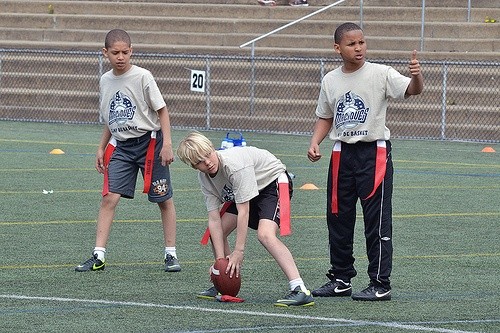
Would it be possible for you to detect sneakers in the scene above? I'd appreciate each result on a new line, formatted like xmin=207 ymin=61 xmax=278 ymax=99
xmin=352 ymin=282 xmax=391 ymax=300
xmin=197 ymin=285 xmax=221 ymax=300
xmin=165 ymin=254 xmax=180 ymax=272
xmin=311 ymin=274 xmax=352 ymax=297
xmin=274 ymin=285 xmax=314 ymax=307
xmin=75 ymin=253 xmax=105 ymax=272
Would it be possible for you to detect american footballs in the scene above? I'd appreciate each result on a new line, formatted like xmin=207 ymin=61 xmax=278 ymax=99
xmin=211 ymin=258 xmax=241 ymax=297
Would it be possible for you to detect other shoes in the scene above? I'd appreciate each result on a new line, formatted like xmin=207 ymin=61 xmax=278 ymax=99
xmin=257 ymin=0 xmax=277 ymax=6
xmin=289 ymin=0 xmax=309 ymax=7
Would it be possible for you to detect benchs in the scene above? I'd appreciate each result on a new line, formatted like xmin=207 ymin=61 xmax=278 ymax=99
xmin=0 ymin=0 xmax=500 ymax=140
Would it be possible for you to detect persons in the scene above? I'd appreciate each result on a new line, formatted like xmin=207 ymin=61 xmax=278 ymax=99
xmin=312 ymin=22 xmax=424 ymax=302
xmin=75 ymin=29 xmax=181 ymax=273
xmin=178 ymin=130 xmax=314 ymax=307
xmin=255 ymin=0 xmax=311 ymax=7
